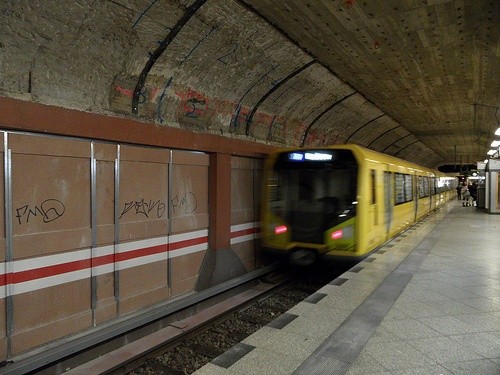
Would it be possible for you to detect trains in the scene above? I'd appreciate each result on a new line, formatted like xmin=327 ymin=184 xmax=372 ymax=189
xmin=262 ymin=144 xmax=460 ymax=267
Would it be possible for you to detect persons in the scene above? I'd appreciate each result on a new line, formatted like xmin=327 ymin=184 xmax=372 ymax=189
xmin=455 ymin=181 xmax=478 ymax=207
